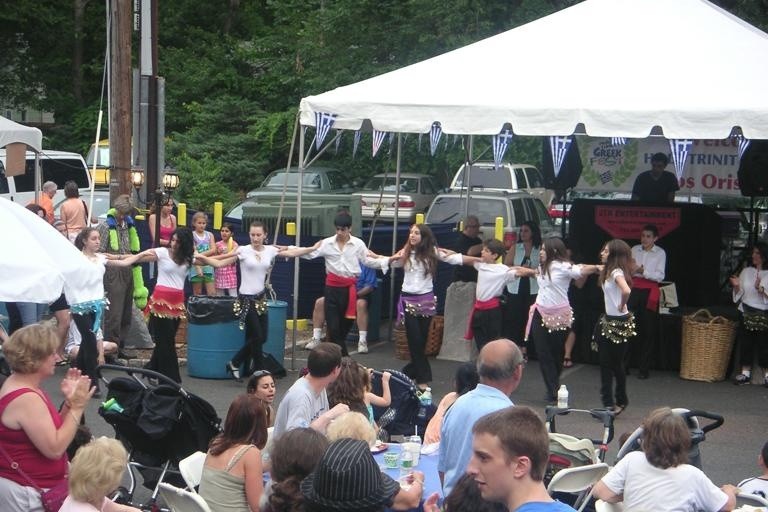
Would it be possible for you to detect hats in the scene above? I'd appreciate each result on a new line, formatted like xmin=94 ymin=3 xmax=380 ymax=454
xmin=300 ymin=439 xmax=400 ymax=510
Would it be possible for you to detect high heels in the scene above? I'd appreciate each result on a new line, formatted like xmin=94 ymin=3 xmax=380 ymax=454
xmin=142 ymin=373 xmax=158 ymax=387
xmin=226 ymin=362 xmax=242 ymax=382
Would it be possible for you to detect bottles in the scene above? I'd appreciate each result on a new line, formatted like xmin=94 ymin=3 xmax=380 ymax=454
xmin=418 ymin=385 xmax=432 ymax=417
xmin=556 ymin=384 xmax=570 ymax=409
xmin=399 ymin=449 xmax=414 ymax=476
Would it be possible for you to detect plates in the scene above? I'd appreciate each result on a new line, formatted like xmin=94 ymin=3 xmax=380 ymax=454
xmin=370 ymin=443 xmax=390 ymax=453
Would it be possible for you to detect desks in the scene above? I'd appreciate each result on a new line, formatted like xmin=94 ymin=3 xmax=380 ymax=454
xmin=356 ymin=439 xmax=448 ymax=511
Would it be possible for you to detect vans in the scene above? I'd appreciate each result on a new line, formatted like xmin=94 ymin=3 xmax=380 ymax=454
xmin=83 ymin=137 xmax=135 ymax=189
xmin=0 ymin=147 xmax=96 ymax=226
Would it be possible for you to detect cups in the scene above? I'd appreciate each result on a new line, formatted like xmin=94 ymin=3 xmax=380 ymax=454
xmin=402 ymin=435 xmax=422 ymax=467
xmin=103 ymin=398 xmax=124 ymax=415
xmin=383 ymin=451 xmax=400 ymax=468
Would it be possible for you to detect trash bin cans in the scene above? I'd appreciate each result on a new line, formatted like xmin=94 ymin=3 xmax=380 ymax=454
xmin=188 ymin=296 xmax=288 ymax=379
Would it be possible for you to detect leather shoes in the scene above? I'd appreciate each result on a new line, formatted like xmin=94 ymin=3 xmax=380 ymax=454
xmin=522 ymin=357 xmax=767 ymax=417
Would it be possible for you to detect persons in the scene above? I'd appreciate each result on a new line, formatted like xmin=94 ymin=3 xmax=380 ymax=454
xmin=624 ymin=225 xmax=666 ymax=379
xmin=730 ymin=245 xmax=767 ymax=385
xmin=196 ymin=214 xmax=635 ymax=411
xmin=1 ymin=322 xmax=767 ymax=511
xmin=631 ymin=152 xmax=680 ymax=202
xmin=26 ymin=179 xmax=241 ymax=396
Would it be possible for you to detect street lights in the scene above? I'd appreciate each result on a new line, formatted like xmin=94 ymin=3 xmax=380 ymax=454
xmin=128 ymin=169 xmax=181 ymax=284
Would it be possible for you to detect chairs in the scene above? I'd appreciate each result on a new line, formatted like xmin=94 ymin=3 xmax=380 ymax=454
xmin=155 ymin=478 xmax=215 ymax=510
xmin=545 ymin=463 xmax=608 ymax=512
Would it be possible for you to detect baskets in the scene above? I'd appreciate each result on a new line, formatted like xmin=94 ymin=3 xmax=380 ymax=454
xmin=390 ymin=315 xmax=444 ymax=359
xmin=680 ymin=308 xmax=737 ymax=382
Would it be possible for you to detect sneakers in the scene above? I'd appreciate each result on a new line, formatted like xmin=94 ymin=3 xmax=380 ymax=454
xmin=357 ymin=341 xmax=368 ymax=353
xmin=305 ymin=339 xmax=320 ymax=350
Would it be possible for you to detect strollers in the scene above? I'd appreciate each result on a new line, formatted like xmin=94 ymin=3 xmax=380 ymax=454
xmin=367 ymin=366 xmax=441 ymax=445
xmin=93 ymin=360 xmax=229 ymax=511
xmin=542 ymin=403 xmax=625 ymax=509
xmin=610 ymin=407 xmax=732 ymax=470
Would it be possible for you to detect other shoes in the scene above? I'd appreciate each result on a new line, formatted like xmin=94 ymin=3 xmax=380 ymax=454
xmin=90 ymin=380 xmax=101 ymax=396
xmin=419 ymin=385 xmax=430 ymax=393
